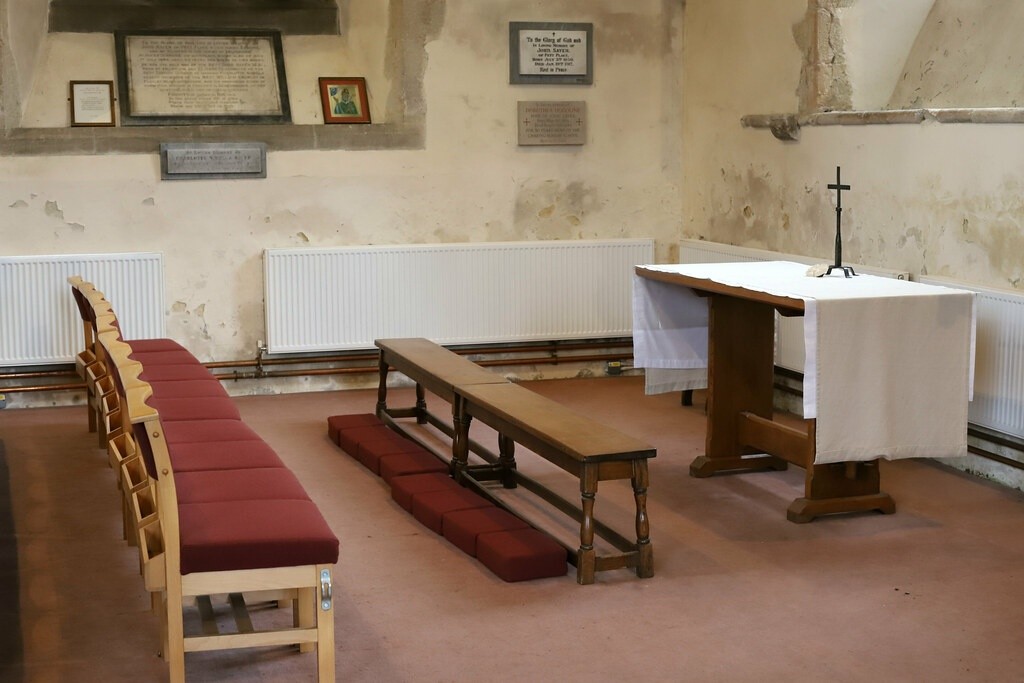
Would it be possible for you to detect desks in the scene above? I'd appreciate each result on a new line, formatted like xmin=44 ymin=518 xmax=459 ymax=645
xmin=631 ymin=260 xmax=976 ymax=524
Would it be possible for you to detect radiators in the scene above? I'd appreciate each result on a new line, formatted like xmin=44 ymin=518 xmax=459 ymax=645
xmin=0 ymin=252 xmax=166 ymax=367
xmin=678 ymin=238 xmax=909 ymax=375
xmin=918 ymin=275 xmax=1024 ymax=439
xmin=262 ymin=238 xmax=655 ymax=353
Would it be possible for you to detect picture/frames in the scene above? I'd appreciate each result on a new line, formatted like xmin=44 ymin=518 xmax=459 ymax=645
xmin=70 ymin=80 xmax=115 ymax=127
xmin=114 ymin=28 xmax=293 ymax=126
xmin=508 ymin=21 xmax=593 ymax=85
xmin=319 ymin=76 xmax=371 ymax=124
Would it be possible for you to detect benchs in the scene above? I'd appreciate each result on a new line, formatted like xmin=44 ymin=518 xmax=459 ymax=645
xmin=374 ymin=338 xmax=656 ymax=586
xmin=68 ymin=273 xmax=341 ymax=683
